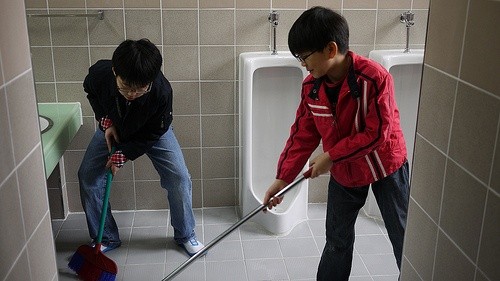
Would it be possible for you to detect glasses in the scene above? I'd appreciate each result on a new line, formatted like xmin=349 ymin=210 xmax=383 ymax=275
xmin=115 ymin=68 xmax=152 ymax=94
xmin=295 ymin=38 xmax=337 ymax=63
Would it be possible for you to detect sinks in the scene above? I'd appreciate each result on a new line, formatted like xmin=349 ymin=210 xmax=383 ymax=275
xmin=39 ymin=116 xmax=49 ymax=132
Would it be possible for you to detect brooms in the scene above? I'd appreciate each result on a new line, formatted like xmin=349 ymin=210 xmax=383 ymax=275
xmin=67 ymin=146 xmax=118 ymax=281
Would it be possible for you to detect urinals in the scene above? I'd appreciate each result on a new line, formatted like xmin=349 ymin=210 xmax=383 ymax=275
xmin=239 ymin=51 xmax=310 ymax=234
xmin=362 ymin=49 xmax=425 ymax=221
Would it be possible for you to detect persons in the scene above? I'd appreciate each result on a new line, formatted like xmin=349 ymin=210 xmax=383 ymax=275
xmin=77 ymin=38 xmax=209 ymax=259
xmin=262 ymin=6 xmax=411 ymax=281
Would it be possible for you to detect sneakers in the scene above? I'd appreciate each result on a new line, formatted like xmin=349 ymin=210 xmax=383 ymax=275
xmin=88 ymin=240 xmax=119 ymax=253
xmin=177 ymin=235 xmax=208 ymax=257
